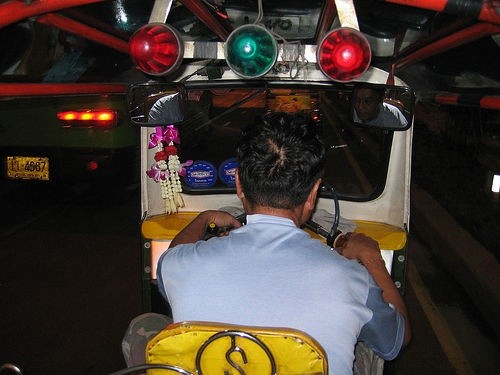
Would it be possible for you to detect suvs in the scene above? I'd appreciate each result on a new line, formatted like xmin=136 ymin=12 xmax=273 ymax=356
xmin=0 ymin=28 xmax=162 ymax=192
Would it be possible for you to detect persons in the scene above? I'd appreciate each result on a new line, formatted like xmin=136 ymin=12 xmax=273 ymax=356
xmin=352 ymin=85 xmax=407 ymax=127
xmin=148 ymin=86 xmax=203 ymax=125
xmin=156 ymin=112 xmax=411 ymax=375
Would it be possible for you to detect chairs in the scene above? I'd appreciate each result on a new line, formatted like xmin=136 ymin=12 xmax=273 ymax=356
xmin=146 ymin=321 xmax=329 ymax=374
